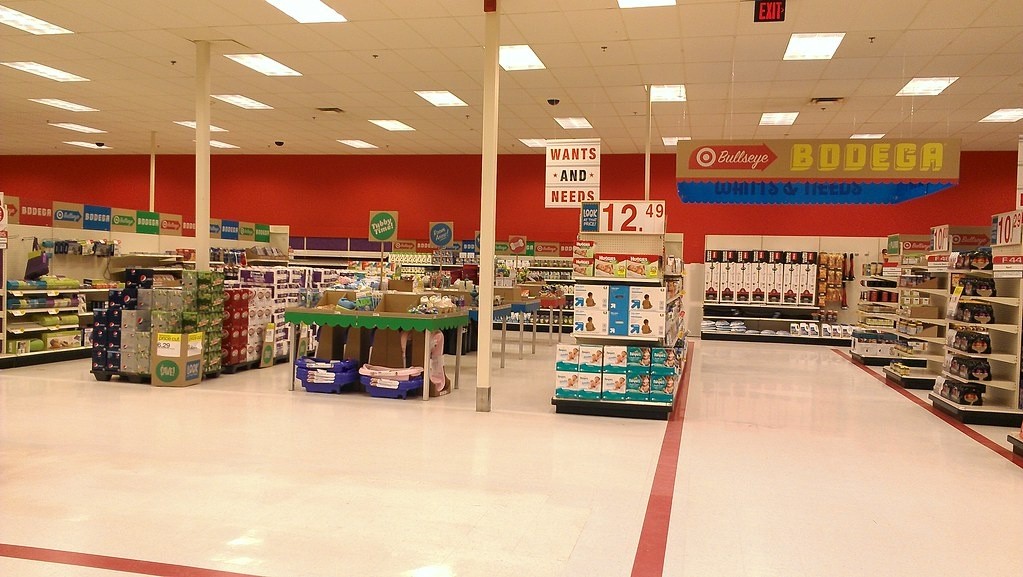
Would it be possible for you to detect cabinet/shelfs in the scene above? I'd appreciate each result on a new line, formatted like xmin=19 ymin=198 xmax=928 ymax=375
xmin=850 ymin=210 xmax=1023 ymax=459
xmin=702 ymin=232 xmax=892 ymax=347
xmin=0 ymin=191 xmax=291 ymax=359
xmin=551 ymin=224 xmax=690 ymax=422
xmin=302 ymin=240 xmax=577 ymax=332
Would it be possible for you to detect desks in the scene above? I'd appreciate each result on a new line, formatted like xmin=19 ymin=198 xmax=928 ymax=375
xmin=282 ymin=281 xmax=568 ymax=401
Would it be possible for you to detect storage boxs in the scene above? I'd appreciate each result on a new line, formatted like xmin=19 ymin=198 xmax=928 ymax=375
xmin=92 ymin=266 xmax=368 ymax=389
xmin=552 ymin=241 xmax=678 ymax=404
xmin=704 ymin=250 xmax=817 ymax=306
